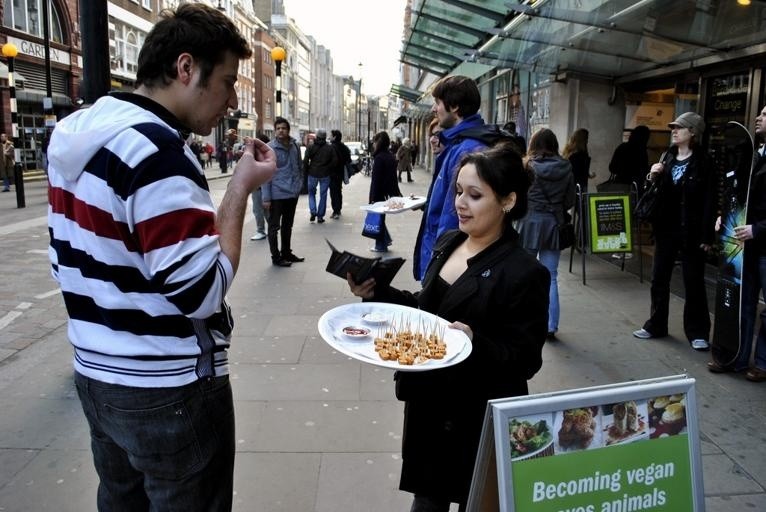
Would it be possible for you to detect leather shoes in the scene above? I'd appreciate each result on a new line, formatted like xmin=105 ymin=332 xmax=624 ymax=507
xmin=273 ymin=259 xmax=292 ymax=267
xmin=283 ymin=254 xmax=304 ymax=261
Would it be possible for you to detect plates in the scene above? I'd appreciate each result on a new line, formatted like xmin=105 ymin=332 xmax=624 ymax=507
xmin=318 ymin=302 xmax=474 ymax=372
xmin=342 ymin=325 xmax=371 ymax=340
xmin=361 ymin=312 xmax=388 ymax=325
xmin=361 ymin=197 xmax=427 ymax=213
xmin=604 ymin=427 xmax=657 ymax=447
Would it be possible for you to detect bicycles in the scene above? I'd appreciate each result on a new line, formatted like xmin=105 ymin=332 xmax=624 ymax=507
xmin=359 ymin=147 xmax=375 ymax=176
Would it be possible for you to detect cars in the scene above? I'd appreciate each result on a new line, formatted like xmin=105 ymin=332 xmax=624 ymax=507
xmin=18 ymin=137 xmax=41 ymax=160
xmin=297 ymin=139 xmax=365 ymax=193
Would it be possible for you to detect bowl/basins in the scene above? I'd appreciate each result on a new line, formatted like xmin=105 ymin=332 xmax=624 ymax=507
xmin=649 ymin=409 xmax=685 ymax=438
xmin=512 ymin=425 xmax=555 ymax=462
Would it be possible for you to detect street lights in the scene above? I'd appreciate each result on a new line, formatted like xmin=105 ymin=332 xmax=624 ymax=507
xmin=1 ymin=41 xmax=29 ymax=209
xmin=272 ymin=44 xmax=284 ymax=121
xmin=357 ymin=58 xmax=365 ymax=139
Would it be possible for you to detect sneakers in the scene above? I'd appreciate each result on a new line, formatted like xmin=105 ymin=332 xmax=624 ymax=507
xmin=691 ymin=339 xmax=709 ymax=350
xmin=369 ymin=241 xmax=392 ymax=252
xmin=611 ymin=253 xmax=633 ymax=260
xmin=250 ymin=233 xmax=266 ymax=240
xmin=310 ymin=213 xmax=339 ymax=223
xmin=707 ymin=360 xmax=748 ymax=374
xmin=633 ymin=327 xmax=652 ymax=339
xmin=745 ymin=366 xmax=766 ymax=382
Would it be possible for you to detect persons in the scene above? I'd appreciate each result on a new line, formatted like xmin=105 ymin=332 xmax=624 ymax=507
xmin=347 ymin=146 xmax=551 ymax=512
xmin=45 ymin=4 xmax=277 ymax=512
xmin=41 ymin=128 xmax=51 ymax=175
xmin=0 ymin=134 xmax=16 ymax=191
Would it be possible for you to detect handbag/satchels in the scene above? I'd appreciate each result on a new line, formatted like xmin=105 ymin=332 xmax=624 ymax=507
xmin=343 ymin=165 xmax=349 ymax=184
xmin=632 ymin=186 xmax=659 ymax=232
xmin=362 ymin=213 xmax=386 ymax=241
xmin=548 ymin=224 xmax=576 ymax=251
xmin=596 ymin=180 xmax=634 ymax=193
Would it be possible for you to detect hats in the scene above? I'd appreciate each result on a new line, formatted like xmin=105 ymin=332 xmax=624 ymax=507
xmin=667 ymin=112 xmax=706 ymax=137
xmin=316 ymin=128 xmax=327 ymax=138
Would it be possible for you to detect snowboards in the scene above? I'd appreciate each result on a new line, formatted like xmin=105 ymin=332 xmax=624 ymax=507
xmin=712 ymin=120 xmax=754 ymax=367
